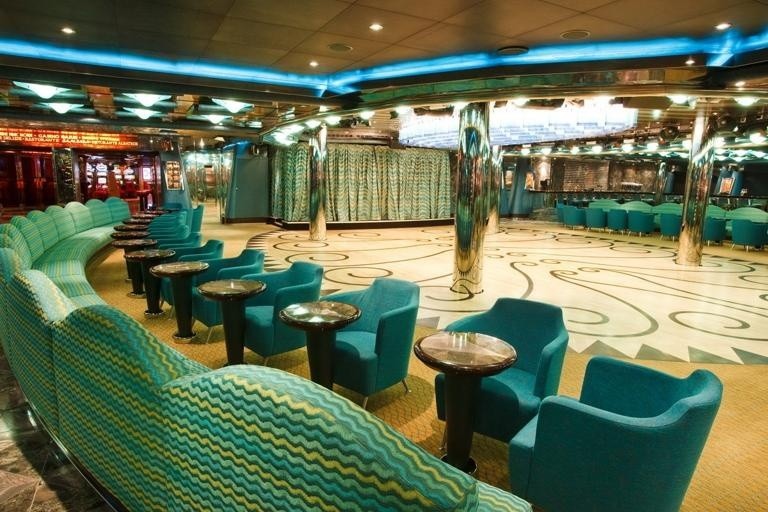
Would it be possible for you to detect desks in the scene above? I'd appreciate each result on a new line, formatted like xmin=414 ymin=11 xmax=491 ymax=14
xmin=276 ymin=299 xmax=361 ymax=400
xmin=412 ymin=326 xmax=515 ymax=481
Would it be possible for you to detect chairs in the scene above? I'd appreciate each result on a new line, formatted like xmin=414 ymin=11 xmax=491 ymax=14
xmin=50 ymin=297 xmax=216 ymax=500
xmin=1 ymin=192 xmax=341 ymax=386
xmin=434 ymin=299 xmax=725 ymax=510
xmin=319 ymin=275 xmax=420 ymax=410
xmin=2 ymin=269 xmax=116 ymax=473
xmin=168 ymin=344 xmax=543 ymax=512
xmin=551 ymin=192 xmax=767 ymax=253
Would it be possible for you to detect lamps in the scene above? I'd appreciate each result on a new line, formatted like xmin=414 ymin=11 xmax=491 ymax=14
xmin=116 ymin=87 xmax=172 ymax=122
xmin=194 ymin=93 xmax=257 ymax=125
xmin=397 ymin=93 xmax=768 ymax=164
xmin=16 ymin=77 xmax=84 ymax=120
xmin=273 ymin=108 xmax=376 ymax=147
